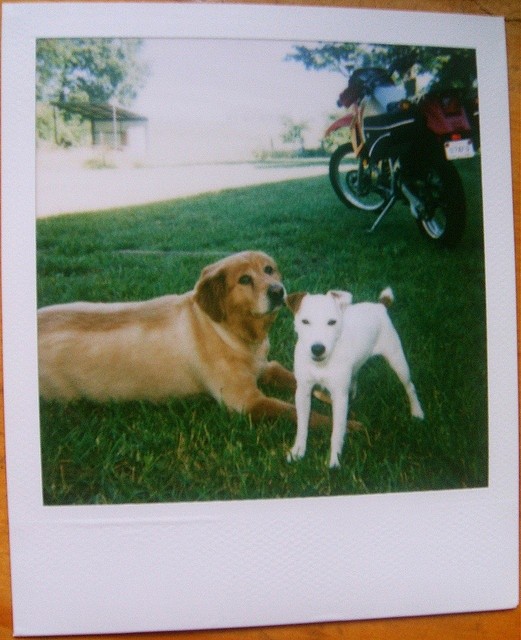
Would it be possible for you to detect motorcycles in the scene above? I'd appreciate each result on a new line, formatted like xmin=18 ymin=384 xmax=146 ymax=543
xmin=324 ymin=50 xmax=476 ymax=241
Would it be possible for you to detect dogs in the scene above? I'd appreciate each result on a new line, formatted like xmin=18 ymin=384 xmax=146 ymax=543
xmin=281 ymin=285 xmax=423 ymax=469
xmin=35 ymin=249 xmax=362 ymax=434
